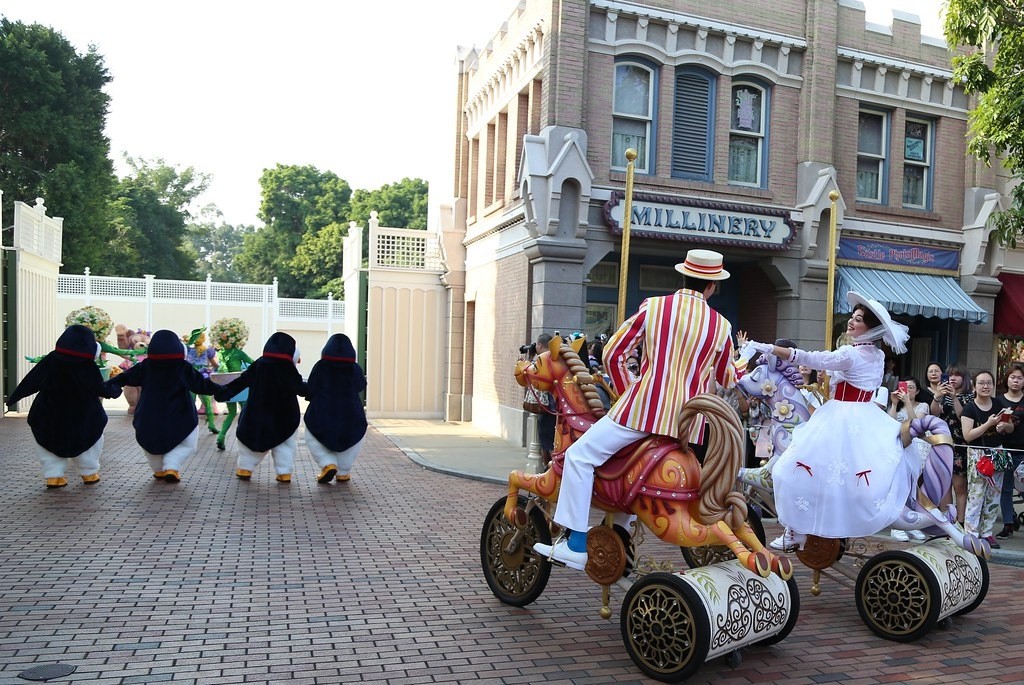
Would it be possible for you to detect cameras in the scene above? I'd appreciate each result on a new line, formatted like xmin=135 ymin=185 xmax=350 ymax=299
xmin=628 ymin=362 xmax=639 ymax=370
xmin=520 ymin=342 xmax=537 ymax=355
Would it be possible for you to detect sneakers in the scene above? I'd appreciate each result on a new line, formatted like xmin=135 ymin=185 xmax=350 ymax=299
xmin=890 ymin=527 xmax=910 ymax=542
xmin=906 ymin=527 xmax=926 ymax=540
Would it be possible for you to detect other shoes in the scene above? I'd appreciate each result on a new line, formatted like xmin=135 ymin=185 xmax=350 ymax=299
xmin=997 ymin=526 xmax=1014 ymax=539
xmin=985 ymin=534 xmax=1000 ymax=550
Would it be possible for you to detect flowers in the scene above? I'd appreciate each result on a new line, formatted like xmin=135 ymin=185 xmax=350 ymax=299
xmin=65 ymin=306 xmax=115 ymax=343
xmin=209 ymin=318 xmax=249 ymax=350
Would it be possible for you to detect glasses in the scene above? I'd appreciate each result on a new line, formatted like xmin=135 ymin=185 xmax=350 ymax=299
xmin=977 ymin=381 xmax=994 ymax=386
xmin=908 ymin=385 xmax=917 ymax=389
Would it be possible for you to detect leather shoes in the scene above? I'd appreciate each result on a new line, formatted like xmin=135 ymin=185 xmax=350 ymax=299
xmin=534 ymin=539 xmax=588 ymax=570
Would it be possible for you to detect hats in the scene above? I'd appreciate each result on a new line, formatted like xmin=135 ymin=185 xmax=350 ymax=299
xmin=676 ymin=250 xmax=729 ymax=280
xmin=847 ymin=291 xmax=910 ymax=355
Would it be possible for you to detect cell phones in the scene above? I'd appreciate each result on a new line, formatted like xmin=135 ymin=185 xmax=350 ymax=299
xmin=941 ymin=372 xmax=949 ymax=386
xmin=898 ymin=381 xmax=908 ymax=394
xmin=996 ymin=409 xmax=1006 ymax=416
xmin=555 ymin=330 xmax=560 ymax=335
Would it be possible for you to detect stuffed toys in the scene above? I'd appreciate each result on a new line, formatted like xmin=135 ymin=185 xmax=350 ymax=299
xmin=215 ymin=332 xmax=307 ymax=481
xmin=6 ymin=324 xmax=123 ymax=489
xmin=114 ymin=324 xmax=152 ymax=414
xmin=303 ymin=333 xmax=368 ymax=482
xmin=108 ymin=329 xmax=230 ymax=484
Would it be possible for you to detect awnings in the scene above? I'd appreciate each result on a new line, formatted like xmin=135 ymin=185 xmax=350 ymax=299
xmin=835 ymin=265 xmax=988 ymax=325
xmin=995 ymin=272 xmax=1024 ymax=344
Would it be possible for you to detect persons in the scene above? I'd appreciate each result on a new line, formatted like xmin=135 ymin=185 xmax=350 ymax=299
xmin=882 ymin=358 xmax=899 ymax=393
xmin=995 ymin=365 xmax=1024 ymax=539
xmin=959 ymin=371 xmax=1014 ymax=550
xmin=887 ymin=377 xmax=929 ymax=541
xmin=915 ymin=363 xmax=947 ymax=404
xmin=525 ymin=331 xmax=643 ymax=473
xmin=531 ymin=248 xmax=738 ymax=570
xmin=740 ymin=290 xmax=922 ymax=550
xmin=930 ymin=363 xmax=978 ymax=528
xmin=688 ymin=330 xmax=825 ymax=469
xmin=24 ymin=325 xmax=255 ymax=451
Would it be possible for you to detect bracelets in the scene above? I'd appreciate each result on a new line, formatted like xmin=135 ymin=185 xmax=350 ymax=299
xmin=933 ymin=395 xmax=942 ymax=402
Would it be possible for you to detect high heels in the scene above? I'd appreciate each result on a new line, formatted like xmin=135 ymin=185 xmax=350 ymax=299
xmin=769 ymin=527 xmax=808 ymax=551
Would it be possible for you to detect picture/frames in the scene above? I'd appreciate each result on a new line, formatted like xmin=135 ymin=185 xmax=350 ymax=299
xmin=586 ymin=303 xmax=617 ymax=342
xmin=639 ymin=264 xmax=685 ymax=291
xmin=587 ymin=261 xmax=618 ymax=288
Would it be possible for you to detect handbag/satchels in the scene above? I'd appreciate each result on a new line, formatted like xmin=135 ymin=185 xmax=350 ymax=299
xmin=985 ymin=449 xmax=1014 ymax=472
